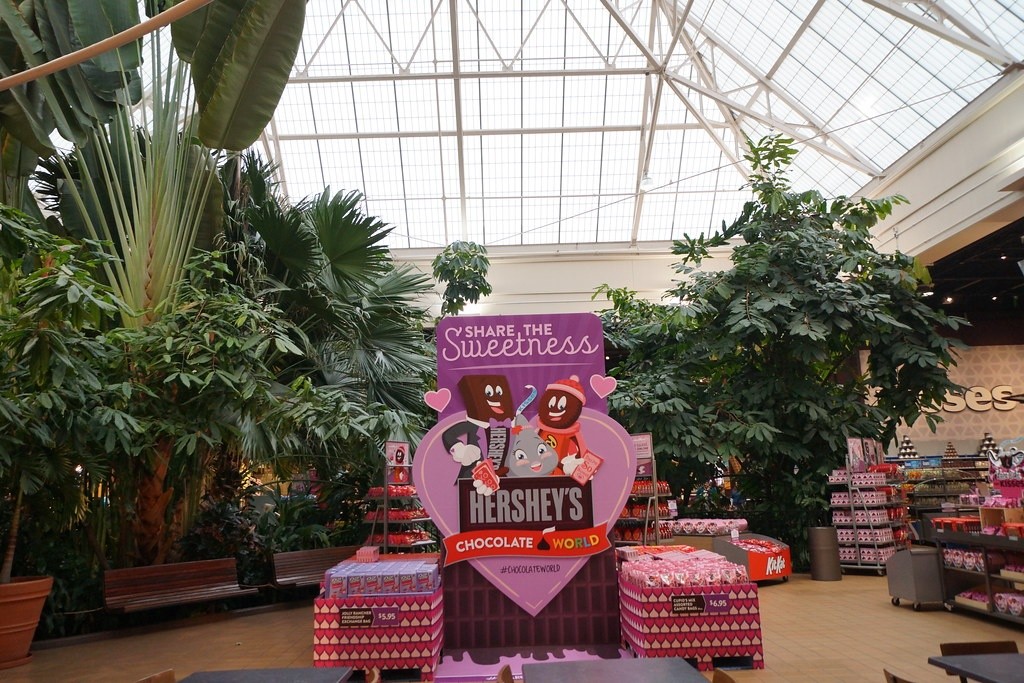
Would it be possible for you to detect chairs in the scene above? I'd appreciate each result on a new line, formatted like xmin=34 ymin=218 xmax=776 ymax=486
xmin=940 ymin=641 xmax=1019 ymax=683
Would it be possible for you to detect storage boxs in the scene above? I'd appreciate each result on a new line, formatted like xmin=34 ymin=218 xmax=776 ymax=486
xmin=325 ymin=547 xmax=441 ymax=598
xmin=829 ymin=463 xmax=895 ymax=561
xmin=979 ymin=507 xmax=1023 ymax=529
xmin=942 ymin=548 xmax=1005 ymax=576
xmin=615 ymin=546 xmax=726 ymax=562
xmin=955 ymin=583 xmax=1024 ymax=616
xmin=1000 ymin=569 xmax=1024 ymax=581
xmin=651 ymin=518 xmax=747 ymax=534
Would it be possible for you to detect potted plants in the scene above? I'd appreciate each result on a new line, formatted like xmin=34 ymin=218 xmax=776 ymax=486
xmin=0 ymin=251 xmax=271 ymax=670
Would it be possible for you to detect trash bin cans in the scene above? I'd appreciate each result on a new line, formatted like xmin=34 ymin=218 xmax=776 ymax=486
xmin=806 ymin=526 xmax=843 ymax=582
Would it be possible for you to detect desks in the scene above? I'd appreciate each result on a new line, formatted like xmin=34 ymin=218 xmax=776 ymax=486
xmin=178 ymin=667 xmax=352 ymax=683
xmin=522 ymin=657 xmax=712 ymax=683
xmin=928 ymin=654 xmax=1024 ymax=683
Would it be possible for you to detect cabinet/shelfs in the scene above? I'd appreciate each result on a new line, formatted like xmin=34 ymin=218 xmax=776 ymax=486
xmin=936 ymin=529 xmax=1024 ymax=624
xmin=827 ymin=455 xmax=891 ymax=575
xmin=887 ymin=455 xmax=989 ymax=544
xmin=673 ymin=531 xmax=752 ymax=551
xmin=313 ymin=585 xmax=446 ymax=683
xmin=614 ymin=452 xmax=673 ymax=546
xmin=615 ymin=570 xmax=764 ymax=671
xmin=363 ymin=465 xmax=436 ymax=554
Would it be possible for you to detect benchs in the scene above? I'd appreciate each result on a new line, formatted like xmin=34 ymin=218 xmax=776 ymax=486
xmin=104 ymin=558 xmax=258 ymax=612
xmin=273 ymin=545 xmax=362 ymax=585
